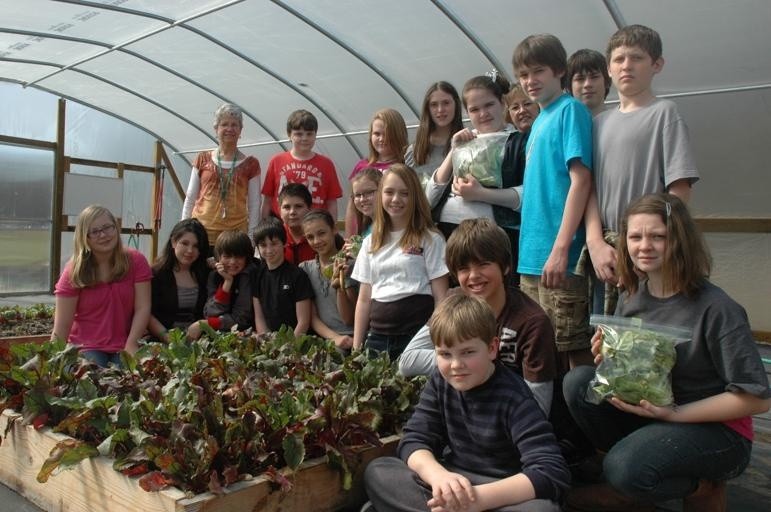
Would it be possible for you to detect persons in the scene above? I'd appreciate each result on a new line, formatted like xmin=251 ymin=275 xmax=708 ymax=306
xmin=361 ymin=293 xmax=571 ymax=511
xmin=563 ymin=194 xmax=771 ymax=511
xmin=19 ymin=204 xmax=152 ymax=369
xmin=145 ymin=26 xmax=699 ymax=457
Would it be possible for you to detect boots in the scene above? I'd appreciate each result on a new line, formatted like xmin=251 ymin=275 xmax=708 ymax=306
xmin=683 ymin=479 xmax=726 ymax=512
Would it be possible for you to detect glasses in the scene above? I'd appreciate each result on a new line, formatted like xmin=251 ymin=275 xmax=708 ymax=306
xmin=87 ymin=224 xmax=116 ymax=238
xmin=351 ymin=190 xmax=376 ymax=201
